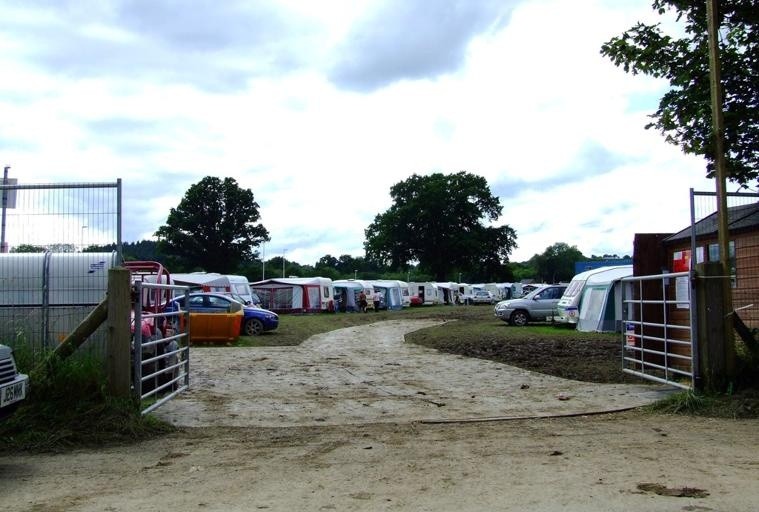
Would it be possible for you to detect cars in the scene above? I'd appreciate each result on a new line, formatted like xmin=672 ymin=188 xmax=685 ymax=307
xmin=473 ymin=291 xmax=495 ymax=305
xmin=494 ymin=285 xmax=568 ymax=326
xmin=161 ymin=293 xmax=279 ymax=336
xmin=0 ymin=344 xmax=31 ymax=423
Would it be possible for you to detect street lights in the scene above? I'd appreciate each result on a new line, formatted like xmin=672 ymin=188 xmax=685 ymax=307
xmin=283 ymin=248 xmax=288 ymax=278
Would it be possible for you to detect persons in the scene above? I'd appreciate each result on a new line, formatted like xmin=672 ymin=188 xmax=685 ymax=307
xmin=360 ymin=289 xmax=367 ymax=313
xmin=372 ymin=289 xmax=383 ymax=311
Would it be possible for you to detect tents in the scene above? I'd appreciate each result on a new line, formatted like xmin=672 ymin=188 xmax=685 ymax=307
xmin=432 ymin=281 xmax=547 ymax=304
xmin=332 ymin=278 xmax=374 ymax=308
xmin=407 ymin=282 xmax=438 ymax=306
xmin=575 ymin=268 xmax=633 ymax=335
xmin=247 ymin=276 xmax=336 ymax=311
xmin=170 ymin=272 xmax=253 ymax=307
xmin=364 ymin=279 xmax=410 ymax=309
